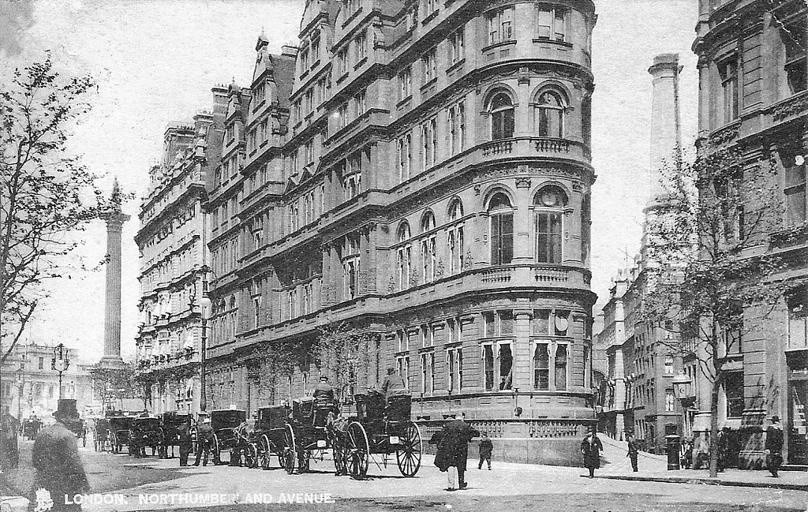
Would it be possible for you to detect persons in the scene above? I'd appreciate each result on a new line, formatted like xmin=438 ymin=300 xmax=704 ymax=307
xmin=581 ymin=428 xmax=603 ymax=479
xmin=476 ymin=431 xmax=492 ymax=470
xmin=676 ymin=425 xmax=739 ymax=472
xmin=433 ymin=410 xmax=480 ymax=491
xmin=191 ymin=416 xmax=214 ymax=466
xmin=0 ymin=395 xmax=91 ymax=512
xmin=762 ymin=415 xmax=785 ymax=478
xmin=176 ymin=419 xmax=194 ymax=466
xmin=626 ymin=433 xmax=639 ymax=472
xmin=382 ymin=366 xmax=406 ymax=413
xmin=140 ymin=409 xmax=148 ymax=418
xmin=312 ymin=374 xmax=335 ymax=429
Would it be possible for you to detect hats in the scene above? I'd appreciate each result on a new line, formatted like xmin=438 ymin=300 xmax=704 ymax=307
xmin=52 ymin=399 xmax=79 ymax=416
xmin=385 ymin=367 xmax=396 ymax=375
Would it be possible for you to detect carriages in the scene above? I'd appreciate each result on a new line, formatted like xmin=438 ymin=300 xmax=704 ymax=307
xmin=21 ymin=417 xmax=43 ymax=441
xmin=68 ymin=416 xmax=88 ymax=448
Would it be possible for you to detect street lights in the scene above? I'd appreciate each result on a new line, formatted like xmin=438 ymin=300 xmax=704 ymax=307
xmin=52 ymin=343 xmax=69 ymax=396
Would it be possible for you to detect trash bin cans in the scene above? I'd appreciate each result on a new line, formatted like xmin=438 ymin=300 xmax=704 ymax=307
xmin=664 ymin=435 xmax=681 ymax=470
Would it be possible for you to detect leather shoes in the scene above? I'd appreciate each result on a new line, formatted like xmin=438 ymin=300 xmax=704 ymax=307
xmin=448 ymin=487 xmax=454 ymax=491
xmin=459 ymin=482 xmax=467 ymax=490
